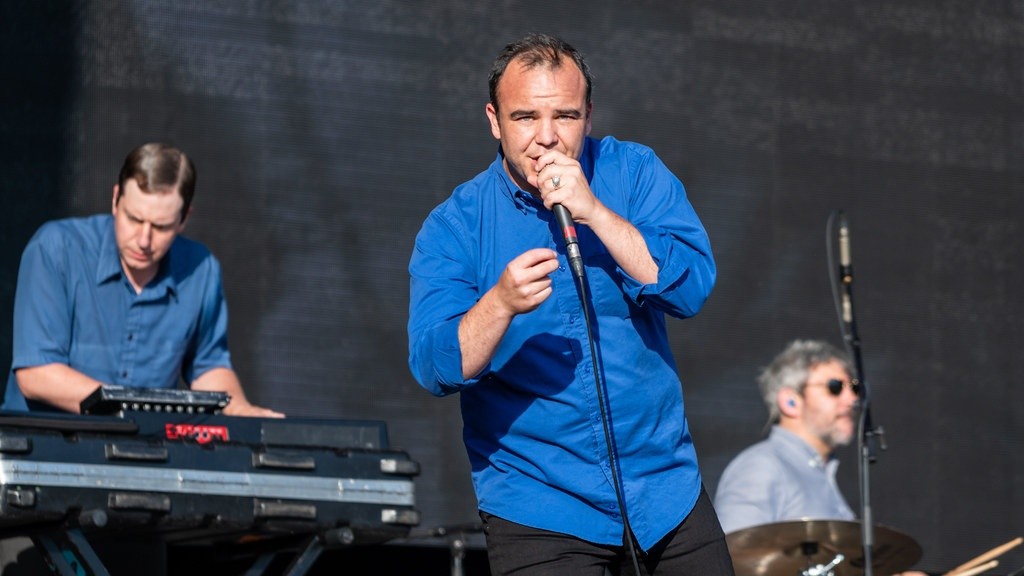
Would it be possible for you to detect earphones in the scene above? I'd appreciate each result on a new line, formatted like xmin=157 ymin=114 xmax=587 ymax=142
xmin=787 ymin=400 xmax=795 ymax=406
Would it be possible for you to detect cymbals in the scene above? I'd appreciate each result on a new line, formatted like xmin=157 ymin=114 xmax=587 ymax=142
xmin=717 ymin=517 xmax=924 ymax=576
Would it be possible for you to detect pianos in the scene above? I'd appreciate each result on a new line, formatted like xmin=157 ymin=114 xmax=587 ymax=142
xmin=1 ymin=383 xmax=424 ymax=544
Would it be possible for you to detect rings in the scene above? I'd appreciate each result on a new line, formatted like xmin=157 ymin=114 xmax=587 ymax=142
xmin=552 ymin=175 xmax=560 ymax=189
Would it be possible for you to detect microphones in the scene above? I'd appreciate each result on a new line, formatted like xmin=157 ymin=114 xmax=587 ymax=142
xmin=538 ymin=163 xmax=584 ymax=279
xmin=839 ymin=226 xmax=855 ymax=340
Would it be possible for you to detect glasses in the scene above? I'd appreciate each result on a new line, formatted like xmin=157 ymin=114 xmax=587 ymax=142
xmin=807 ymin=379 xmax=861 ymax=395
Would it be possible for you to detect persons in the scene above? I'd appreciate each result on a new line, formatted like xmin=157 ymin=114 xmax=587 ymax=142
xmin=0 ymin=141 xmax=285 ymax=418
xmin=713 ymin=339 xmax=928 ymax=576
xmin=407 ymin=34 xmax=735 ymax=576
